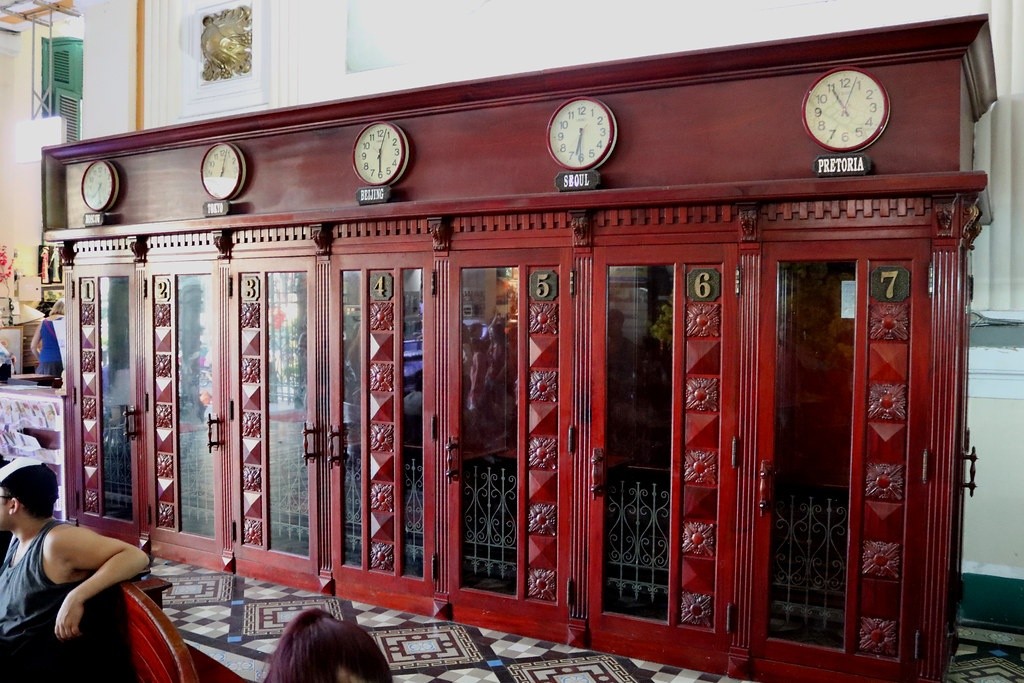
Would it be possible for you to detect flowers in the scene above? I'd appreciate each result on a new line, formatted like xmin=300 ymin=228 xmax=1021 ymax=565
xmin=0 ymin=252 xmax=15 ymax=296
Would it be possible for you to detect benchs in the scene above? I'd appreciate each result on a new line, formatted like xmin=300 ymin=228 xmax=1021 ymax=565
xmin=120 ymin=581 xmax=250 ymax=683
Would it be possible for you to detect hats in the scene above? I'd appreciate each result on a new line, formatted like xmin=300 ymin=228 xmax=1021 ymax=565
xmin=0 ymin=457 xmax=60 ymax=516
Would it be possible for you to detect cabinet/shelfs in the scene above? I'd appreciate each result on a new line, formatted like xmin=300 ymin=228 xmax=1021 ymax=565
xmin=42 ymin=14 xmax=999 ymax=683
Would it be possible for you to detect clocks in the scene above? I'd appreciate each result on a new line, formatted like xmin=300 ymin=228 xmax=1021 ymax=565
xmin=352 ymin=121 xmax=409 ymax=186
xmin=545 ymin=97 xmax=617 ymax=171
xmin=80 ymin=160 xmax=118 ymax=211
xmin=200 ymin=143 xmax=246 ymax=199
xmin=801 ymin=66 xmax=891 ymax=153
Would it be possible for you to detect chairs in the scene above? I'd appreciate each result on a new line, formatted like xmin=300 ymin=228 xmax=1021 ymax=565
xmin=0 ymin=532 xmax=119 ymax=683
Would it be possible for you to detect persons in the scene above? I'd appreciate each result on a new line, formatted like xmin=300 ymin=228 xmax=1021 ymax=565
xmin=263 ymin=608 xmax=393 ymax=683
xmin=461 ymin=314 xmax=507 ymax=445
xmin=0 ymin=465 xmax=148 ymax=650
xmin=0 ymin=344 xmax=12 ymax=381
xmin=30 ymin=299 xmax=64 ymax=377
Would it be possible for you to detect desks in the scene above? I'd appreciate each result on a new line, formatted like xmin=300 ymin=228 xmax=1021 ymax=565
xmin=134 ymin=576 xmax=172 ymax=610
